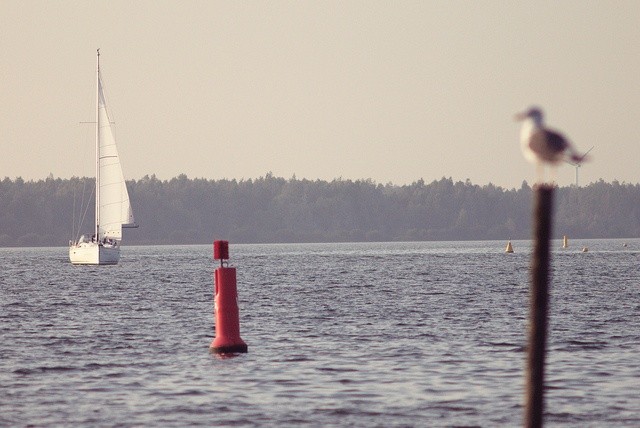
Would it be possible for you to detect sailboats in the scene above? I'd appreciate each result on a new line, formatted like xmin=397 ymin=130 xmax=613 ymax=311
xmin=69 ymin=47 xmax=140 ymax=265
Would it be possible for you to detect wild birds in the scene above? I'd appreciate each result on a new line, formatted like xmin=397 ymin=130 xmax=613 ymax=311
xmin=513 ymin=104 xmax=593 ymax=185
